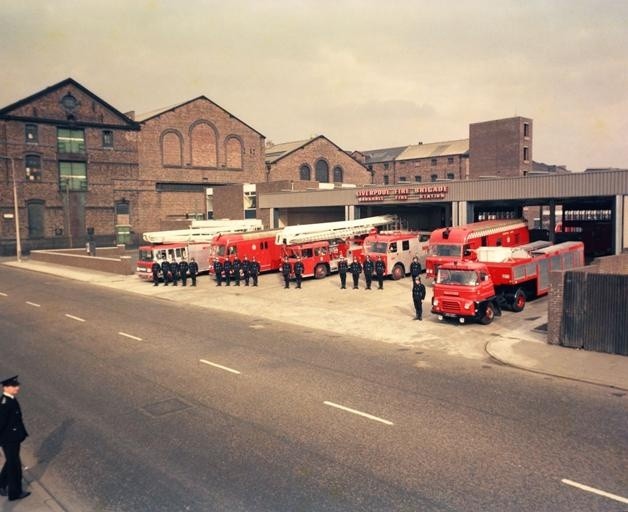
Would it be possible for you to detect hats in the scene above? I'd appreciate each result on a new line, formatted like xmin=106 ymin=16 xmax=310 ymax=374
xmin=0 ymin=374 xmax=20 ymax=386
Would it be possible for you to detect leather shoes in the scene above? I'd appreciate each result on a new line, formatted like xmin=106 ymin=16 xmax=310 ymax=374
xmin=0 ymin=487 xmax=8 ymax=495
xmin=9 ymin=491 xmax=30 ymax=501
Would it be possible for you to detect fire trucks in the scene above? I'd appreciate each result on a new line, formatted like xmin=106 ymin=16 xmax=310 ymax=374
xmin=360 ymin=229 xmax=433 ymax=281
xmin=422 ymin=219 xmax=529 ymax=293
xmin=137 ymin=214 xmax=405 ymax=283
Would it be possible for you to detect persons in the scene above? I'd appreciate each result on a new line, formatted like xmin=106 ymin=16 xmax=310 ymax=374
xmin=214 ymin=255 xmax=261 ymax=286
xmin=0 ymin=374 xmax=32 ymax=501
xmin=413 ymin=276 xmax=426 ymax=321
xmin=410 ymin=256 xmax=421 ymax=285
xmin=151 ymin=256 xmax=198 ymax=287
xmin=338 ymin=254 xmax=386 ymax=290
xmin=293 ymin=256 xmax=304 ymax=289
xmin=281 ymin=256 xmax=292 ymax=288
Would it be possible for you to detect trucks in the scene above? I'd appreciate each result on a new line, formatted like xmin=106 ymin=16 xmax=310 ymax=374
xmin=429 ymin=240 xmax=585 ymax=325
